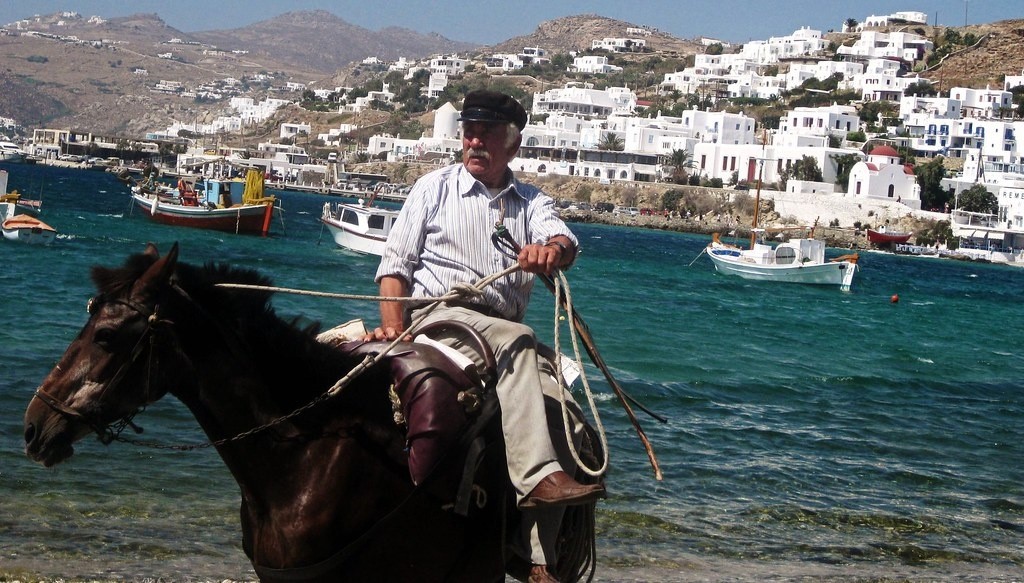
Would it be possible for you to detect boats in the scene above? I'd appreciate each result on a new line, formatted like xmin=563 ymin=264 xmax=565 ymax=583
xmin=2 ymin=213 xmax=59 ymax=245
xmin=864 ymin=228 xmax=913 ymax=245
xmin=0 ymin=140 xmax=42 ymax=165
xmin=321 ymin=203 xmax=401 ymax=257
xmin=115 ymin=170 xmax=275 ymax=237
xmin=706 ymin=129 xmax=859 ymax=293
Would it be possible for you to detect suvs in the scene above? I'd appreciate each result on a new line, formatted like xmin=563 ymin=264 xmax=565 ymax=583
xmin=88 ymin=157 xmax=104 ymax=164
xmin=349 ymin=181 xmax=361 ymax=190
xmin=337 ymin=179 xmax=349 ymax=189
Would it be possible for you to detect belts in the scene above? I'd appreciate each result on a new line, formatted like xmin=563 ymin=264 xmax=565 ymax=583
xmin=446 ymin=299 xmax=508 ymax=320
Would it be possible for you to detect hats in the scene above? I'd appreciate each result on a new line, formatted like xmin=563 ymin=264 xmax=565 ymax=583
xmin=457 ymin=90 xmax=527 ymax=133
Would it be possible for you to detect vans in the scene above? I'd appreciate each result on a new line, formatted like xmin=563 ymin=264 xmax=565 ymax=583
xmin=594 ymin=203 xmax=614 ymax=212
xmin=328 ymin=152 xmax=337 ymax=162
xmin=101 ymin=157 xmax=119 ymax=165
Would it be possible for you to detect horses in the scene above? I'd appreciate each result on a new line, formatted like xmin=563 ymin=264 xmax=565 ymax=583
xmin=20 ymin=239 xmax=610 ymax=583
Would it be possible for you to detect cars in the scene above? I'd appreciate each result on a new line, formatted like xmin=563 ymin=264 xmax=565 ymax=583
xmin=641 ymin=208 xmax=656 ymax=215
xmin=614 ymin=206 xmax=630 ymax=215
xmin=59 ymin=154 xmax=91 ymax=163
xmin=371 ymin=182 xmax=412 ymax=193
xmin=628 ymin=207 xmax=639 ymax=217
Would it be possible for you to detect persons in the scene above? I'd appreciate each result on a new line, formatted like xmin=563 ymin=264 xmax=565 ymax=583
xmin=358 ymin=90 xmax=605 ymax=583
xmin=662 ymin=207 xmax=741 ymax=226
xmin=149 ymin=181 xmax=159 ymax=190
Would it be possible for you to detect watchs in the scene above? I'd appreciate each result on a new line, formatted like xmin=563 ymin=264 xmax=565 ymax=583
xmin=545 ymin=241 xmax=567 ymax=262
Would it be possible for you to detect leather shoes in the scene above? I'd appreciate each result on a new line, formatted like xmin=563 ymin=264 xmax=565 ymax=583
xmin=528 ymin=564 xmax=562 ymax=583
xmin=519 ymin=472 xmax=605 ymax=508
